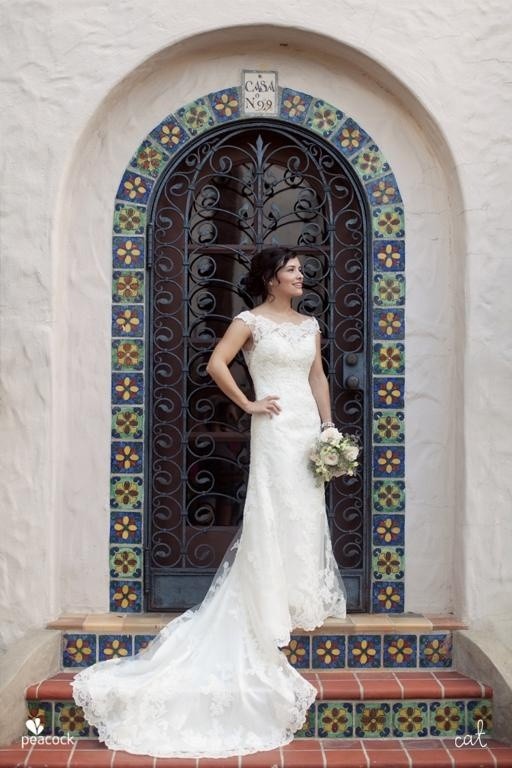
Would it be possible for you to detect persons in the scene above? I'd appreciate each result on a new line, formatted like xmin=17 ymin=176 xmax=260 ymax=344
xmin=68 ymin=247 xmax=348 ymax=759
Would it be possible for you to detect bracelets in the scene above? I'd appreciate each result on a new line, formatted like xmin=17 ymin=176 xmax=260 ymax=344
xmin=321 ymin=422 xmax=336 ymax=428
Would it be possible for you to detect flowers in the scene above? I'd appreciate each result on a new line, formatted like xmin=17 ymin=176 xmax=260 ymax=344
xmin=309 ymin=427 xmax=363 ymax=488
